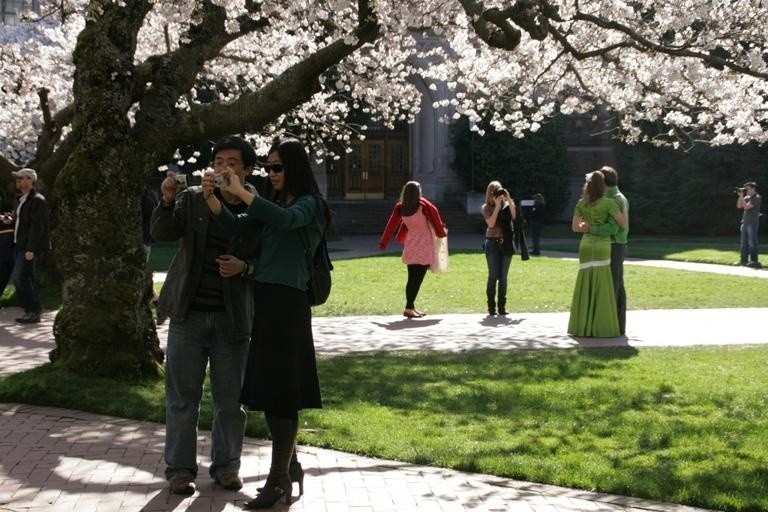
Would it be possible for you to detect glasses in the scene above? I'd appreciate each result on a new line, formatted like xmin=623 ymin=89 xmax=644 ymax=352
xmin=263 ymin=163 xmax=284 ymax=173
xmin=15 ymin=176 xmax=24 ymax=180
xmin=210 ymin=159 xmax=237 ymax=169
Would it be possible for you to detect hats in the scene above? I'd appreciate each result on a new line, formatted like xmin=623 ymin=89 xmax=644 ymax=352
xmin=10 ymin=167 xmax=38 ymax=183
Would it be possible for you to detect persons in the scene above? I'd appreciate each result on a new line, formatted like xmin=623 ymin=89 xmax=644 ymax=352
xmin=530 ymin=193 xmax=545 ymax=256
xmin=481 ymin=182 xmax=516 ymax=316
xmin=1 ymin=167 xmax=48 ymax=323
xmin=736 ymin=182 xmax=766 ymax=267
xmin=568 ymin=173 xmax=626 ymax=339
xmin=201 ymin=134 xmax=332 ymax=509
xmin=577 ymin=167 xmax=629 ymax=334
xmin=379 ymin=180 xmax=448 ymax=319
xmin=150 ymin=137 xmax=259 ymax=496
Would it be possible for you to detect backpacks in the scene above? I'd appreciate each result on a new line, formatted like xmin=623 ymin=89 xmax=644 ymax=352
xmin=269 ymin=189 xmax=335 ymax=306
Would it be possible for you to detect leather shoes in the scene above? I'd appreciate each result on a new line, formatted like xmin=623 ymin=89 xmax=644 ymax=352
xmin=213 ymin=469 xmax=242 ymax=491
xmin=404 ymin=311 xmax=423 ymax=318
xmin=15 ymin=311 xmax=40 ymax=324
xmin=414 ymin=310 xmax=426 ymax=316
xmin=169 ymin=475 xmax=198 ymax=495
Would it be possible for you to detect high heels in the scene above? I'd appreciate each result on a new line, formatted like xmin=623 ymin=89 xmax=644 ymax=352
xmin=256 ymin=463 xmax=305 ymax=495
xmin=243 ymin=478 xmax=293 ymax=510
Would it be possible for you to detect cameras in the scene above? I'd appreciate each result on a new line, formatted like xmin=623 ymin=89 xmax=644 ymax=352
xmin=3 ymin=212 xmax=14 ymax=220
xmin=174 ymin=172 xmax=203 ymax=188
xmin=213 ymin=173 xmax=228 ymax=189
xmin=491 ymin=189 xmax=505 ymax=198
xmin=733 ymin=186 xmax=746 ymax=197
